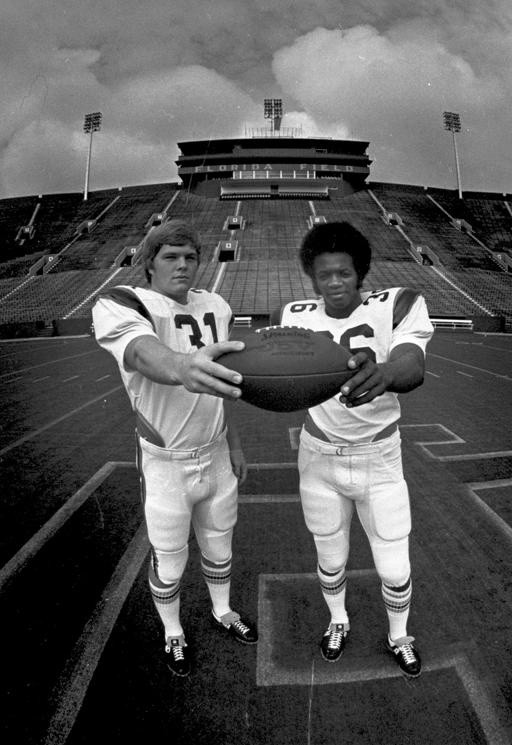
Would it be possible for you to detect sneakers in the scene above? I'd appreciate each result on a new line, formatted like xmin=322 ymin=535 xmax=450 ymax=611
xmin=161 ymin=631 xmax=192 ymax=678
xmin=385 ymin=632 xmax=423 ymax=678
xmin=209 ymin=607 xmax=259 ymax=644
xmin=319 ymin=620 xmax=350 ymax=662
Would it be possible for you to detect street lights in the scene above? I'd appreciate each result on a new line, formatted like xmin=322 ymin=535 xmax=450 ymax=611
xmin=264 ymin=99 xmax=282 ymax=135
xmin=84 ymin=112 xmax=103 ymax=200
xmin=441 ymin=111 xmax=463 ymax=199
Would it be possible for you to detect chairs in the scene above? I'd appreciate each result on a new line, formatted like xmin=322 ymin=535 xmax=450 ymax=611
xmin=0 ymin=183 xmax=512 ymax=330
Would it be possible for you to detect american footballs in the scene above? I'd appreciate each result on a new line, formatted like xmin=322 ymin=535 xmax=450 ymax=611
xmin=210 ymin=326 xmax=360 ymax=413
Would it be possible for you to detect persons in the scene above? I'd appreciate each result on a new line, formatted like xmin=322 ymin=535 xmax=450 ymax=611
xmin=90 ymin=219 xmax=260 ymax=678
xmin=268 ymin=219 xmax=436 ymax=680
